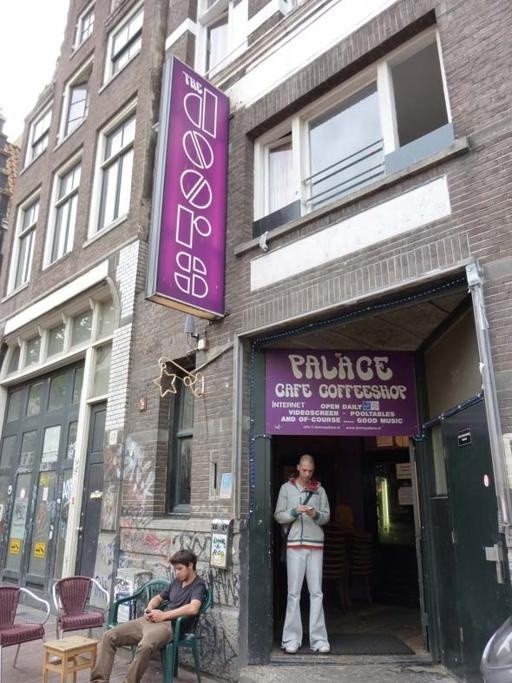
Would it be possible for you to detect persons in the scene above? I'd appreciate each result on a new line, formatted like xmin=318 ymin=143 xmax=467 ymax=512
xmin=274 ymin=454 xmax=331 ymax=654
xmin=89 ymin=549 xmax=206 ymax=682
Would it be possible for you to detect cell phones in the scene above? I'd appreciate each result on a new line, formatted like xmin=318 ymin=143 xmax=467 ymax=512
xmin=309 ymin=506 xmax=314 ymax=510
xmin=146 ymin=609 xmax=151 ymax=614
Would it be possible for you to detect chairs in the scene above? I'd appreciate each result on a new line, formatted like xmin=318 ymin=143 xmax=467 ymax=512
xmin=1 ymin=572 xmax=212 ymax=682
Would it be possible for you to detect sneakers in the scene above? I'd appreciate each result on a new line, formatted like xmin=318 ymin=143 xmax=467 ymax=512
xmin=318 ymin=646 xmax=330 ymax=653
xmin=286 ymin=647 xmax=297 ymax=654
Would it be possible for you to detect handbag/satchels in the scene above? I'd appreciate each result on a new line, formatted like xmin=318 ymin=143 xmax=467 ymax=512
xmin=279 ymin=536 xmax=287 ymax=563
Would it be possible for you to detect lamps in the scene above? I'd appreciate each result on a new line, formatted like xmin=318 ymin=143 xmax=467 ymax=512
xmin=184 ymin=315 xmax=208 ymax=349
